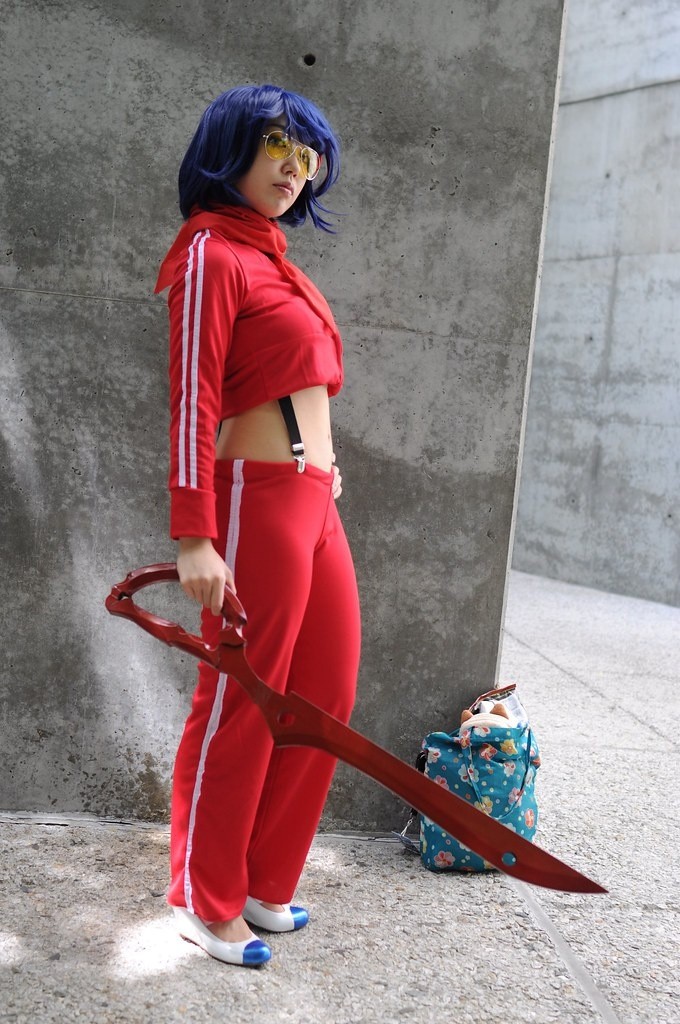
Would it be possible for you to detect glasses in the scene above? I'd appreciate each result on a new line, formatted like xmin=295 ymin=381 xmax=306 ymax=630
xmin=262 ymin=130 xmax=322 ymax=181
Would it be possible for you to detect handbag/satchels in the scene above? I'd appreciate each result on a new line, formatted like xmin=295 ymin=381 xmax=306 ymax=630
xmin=391 ymin=684 xmax=541 ymax=874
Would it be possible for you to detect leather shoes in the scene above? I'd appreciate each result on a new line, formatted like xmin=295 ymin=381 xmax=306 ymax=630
xmin=172 ymin=905 xmax=271 ymax=967
xmin=241 ymin=894 xmax=309 ymax=932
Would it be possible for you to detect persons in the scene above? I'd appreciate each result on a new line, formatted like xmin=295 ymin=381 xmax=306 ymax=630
xmin=153 ymin=85 xmax=361 ymax=965
